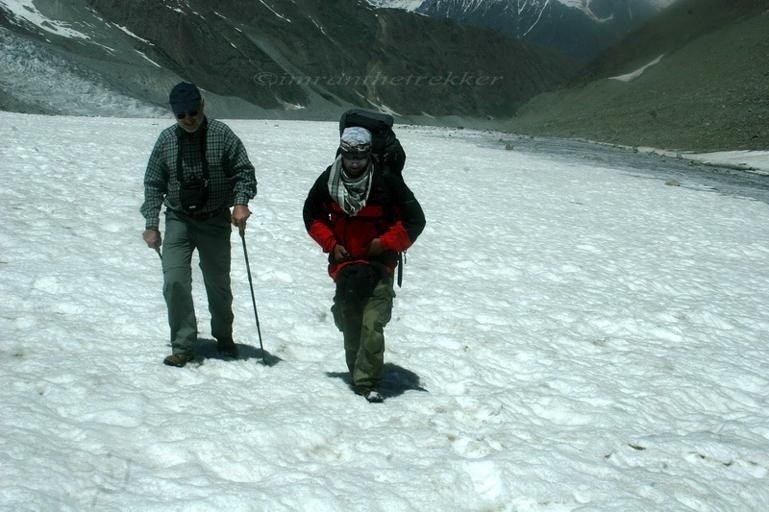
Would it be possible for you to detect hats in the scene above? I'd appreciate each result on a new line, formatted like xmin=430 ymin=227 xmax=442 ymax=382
xmin=169 ymin=82 xmax=201 ymax=113
xmin=341 ymin=127 xmax=373 ymax=152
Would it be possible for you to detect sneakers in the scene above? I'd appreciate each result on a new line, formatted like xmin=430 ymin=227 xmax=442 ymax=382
xmin=163 ymin=351 xmax=194 ymax=367
xmin=354 ymin=385 xmax=383 ymax=403
xmin=216 ymin=336 xmax=239 ymax=357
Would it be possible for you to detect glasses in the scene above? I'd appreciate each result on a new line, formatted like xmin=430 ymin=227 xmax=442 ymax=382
xmin=176 ymin=110 xmax=198 ymax=119
xmin=341 ymin=150 xmax=369 ymax=160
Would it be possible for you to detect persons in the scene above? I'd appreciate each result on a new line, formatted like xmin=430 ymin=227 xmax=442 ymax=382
xmin=139 ymin=83 xmax=257 ymax=366
xmin=302 ymin=124 xmax=427 ymax=406
